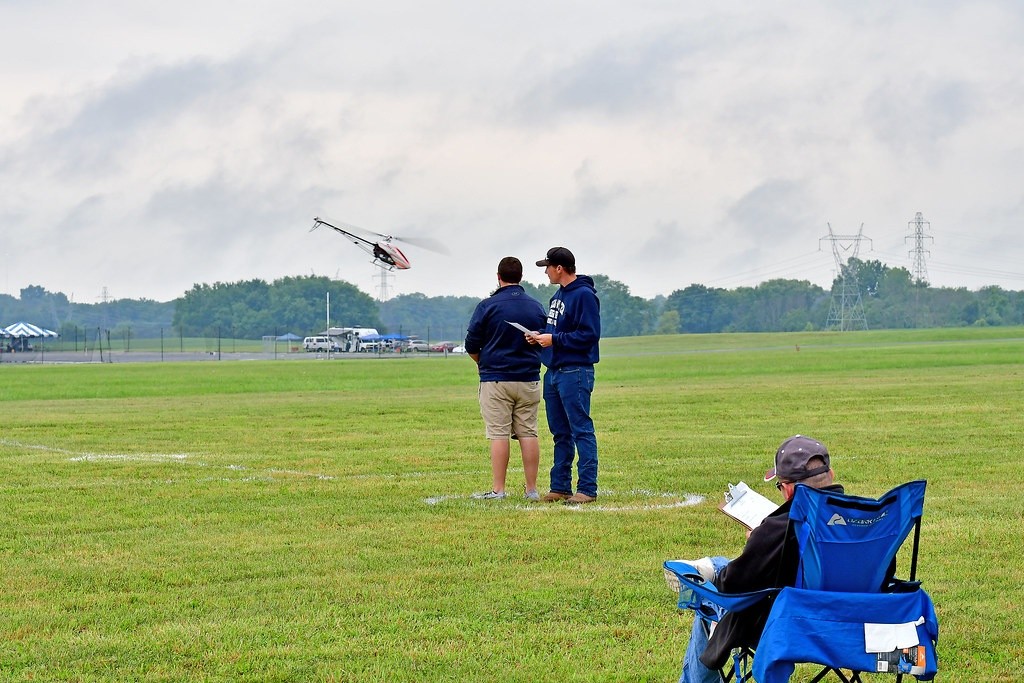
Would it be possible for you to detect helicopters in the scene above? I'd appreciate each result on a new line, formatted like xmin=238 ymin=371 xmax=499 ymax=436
xmin=309 ymin=217 xmax=448 ymax=272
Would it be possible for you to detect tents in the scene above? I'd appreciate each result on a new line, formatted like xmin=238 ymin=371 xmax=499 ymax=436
xmin=0 ymin=321 xmax=61 ymax=351
xmin=360 ymin=333 xmax=408 ymax=352
xmin=277 ymin=332 xmax=303 ymax=353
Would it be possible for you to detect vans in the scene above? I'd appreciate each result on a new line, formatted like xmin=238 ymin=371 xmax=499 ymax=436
xmin=303 ymin=337 xmax=335 ymax=352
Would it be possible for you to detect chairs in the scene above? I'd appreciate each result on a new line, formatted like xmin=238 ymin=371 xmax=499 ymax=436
xmin=663 ymin=480 xmax=938 ymax=683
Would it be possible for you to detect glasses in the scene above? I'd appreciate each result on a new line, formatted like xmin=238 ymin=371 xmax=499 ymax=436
xmin=776 ymin=480 xmax=796 ymax=491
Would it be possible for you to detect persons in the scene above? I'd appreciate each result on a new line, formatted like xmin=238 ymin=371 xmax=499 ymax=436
xmin=663 ymin=434 xmax=897 ymax=683
xmin=525 ymin=247 xmax=601 ymax=504
xmin=381 ymin=339 xmax=386 ymax=352
xmin=334 ymin=341 xmax=338 ymax=351
xmin=28 ymin=342 xmax=32 ymax=351
xmin=346 ymin=340 xmax=350 ymax=352
xmin=465 ymin=256 xmax=547 ymax=502
xmin=7 ymin=343 xmax=10 ymax=352
xmin=356 ymin=338 xmax=359 ymax=352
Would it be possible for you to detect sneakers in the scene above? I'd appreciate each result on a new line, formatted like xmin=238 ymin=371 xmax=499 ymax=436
xmin=477 ymin=490 xmax=506 ymax=499
xmin=664 ymin=557 xmax=715 ymax=592
xmin=565 ymin=492 xmax=596 ymax=503
xmin=544 ymin=491 xmax=572 ymax=501
xmin=522 ymin=491 xmax=540 ymax=500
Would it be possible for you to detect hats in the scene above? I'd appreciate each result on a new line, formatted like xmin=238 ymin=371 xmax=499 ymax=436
xmin=763 ymin=435 xmax=831 ymax=481
xmin=536 ymin=247 xmax=575 ymax=267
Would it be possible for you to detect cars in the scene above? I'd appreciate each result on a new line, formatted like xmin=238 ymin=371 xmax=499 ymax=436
xmin=401 ymin=340 xmax=434 ymax=352
xmin=432 ymin=342 xmax=459 ymax=353
xmin=452 ymin=342 xmax=467 ymax=353
